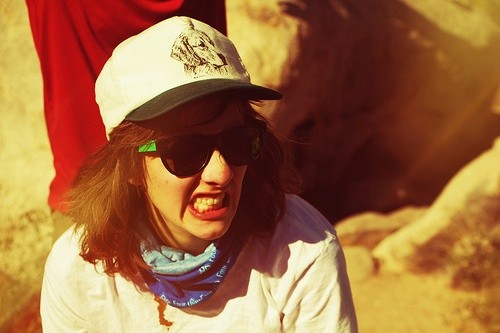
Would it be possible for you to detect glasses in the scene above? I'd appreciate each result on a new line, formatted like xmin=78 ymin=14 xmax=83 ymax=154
xmin=136 ymin=118 xmax=267 ymax=177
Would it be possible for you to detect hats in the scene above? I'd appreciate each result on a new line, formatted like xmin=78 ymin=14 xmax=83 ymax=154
xmin=95 ymin=15 xmax=284 ymax=142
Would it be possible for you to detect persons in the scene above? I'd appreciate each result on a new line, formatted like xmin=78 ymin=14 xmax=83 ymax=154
xmin=40 ymin=16 xmax=358 ymax=333
